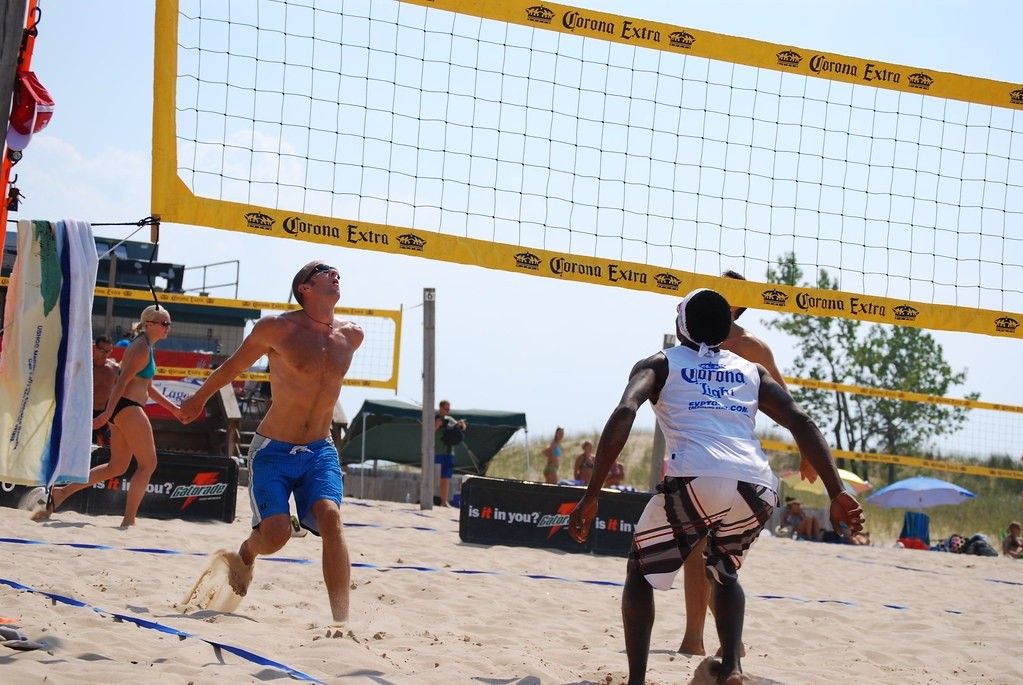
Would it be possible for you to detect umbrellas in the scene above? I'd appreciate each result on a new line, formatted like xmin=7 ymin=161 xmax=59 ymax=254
xmin=784 ymin=469 xmax=873 ymax=496
xmin=865 ymin=475 xmax=977 ymax=513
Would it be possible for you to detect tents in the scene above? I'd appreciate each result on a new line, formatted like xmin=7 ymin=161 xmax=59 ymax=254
xmin=340 ymin=399 xmax=531 ymax=499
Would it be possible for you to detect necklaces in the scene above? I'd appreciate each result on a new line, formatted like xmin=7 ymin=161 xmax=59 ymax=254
xmin=304 ymin=311 xmax=333 ymax=329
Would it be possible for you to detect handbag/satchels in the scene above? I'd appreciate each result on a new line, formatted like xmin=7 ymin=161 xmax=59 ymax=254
xmin=441 ymin=418 xmax=464 ymax=454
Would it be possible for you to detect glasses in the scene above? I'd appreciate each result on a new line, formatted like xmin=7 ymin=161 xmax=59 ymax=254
xmin=97 ymin=345 xmax=113 ymax=355
xmin=146 ymin=320 xmax=171 ymax=326
xmin=301 ymin=264 xmax=340 ymax=297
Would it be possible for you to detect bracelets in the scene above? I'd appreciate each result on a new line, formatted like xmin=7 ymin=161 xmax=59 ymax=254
xmin=831 ymin=491 xmax=846 ymax=501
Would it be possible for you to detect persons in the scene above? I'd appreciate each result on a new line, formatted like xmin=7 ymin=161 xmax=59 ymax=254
xmin=781 ymin=498 xmax=821 ymax=542
xmin=92 ymin=335 xmax=119 ymax=447
xmin=116 ymin=332 xmax=132 ymax=346
xmin=575 ymin=441 xmax=595 ymax=486
xmin=1002 ymin=522 xmax=1023 ymax=558
xmin=180 ymin=260 xmax=364 ymax=629
xmin=840 ymin=522 xmax=870 ymax=545
xmin=679 ymin=270 xmax=819 ymax=657
xmin=543 ymin=428 xmax=564 ymax=483
xmin=663 ymin=459 xmax=669 ymax=477
xmin=46 ymin=305 xmax=182 ymax=531
xmin=568 ymin=289 xmax=865 ymax=685
xmin=931 ymin=534 xmax=988 ymax=553
xmin=603 ymin=459 xmax=624 ymax=488
xmin=435 ymin=400 xmax=465 ymax=507
